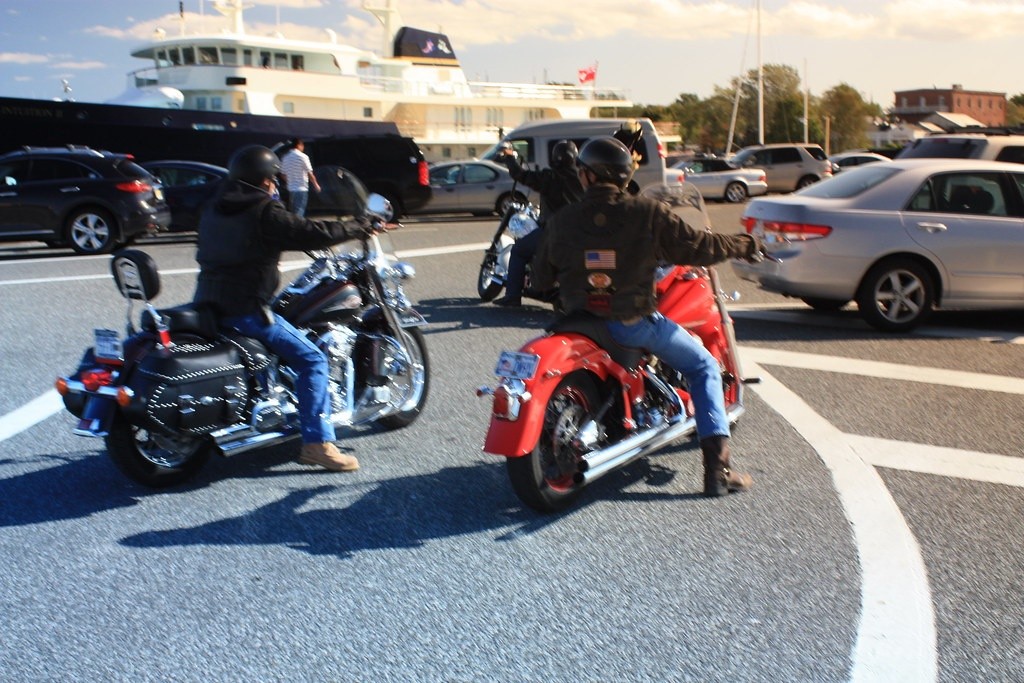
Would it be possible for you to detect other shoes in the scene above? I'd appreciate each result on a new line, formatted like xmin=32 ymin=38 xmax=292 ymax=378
xmin=493 ymin=295 xmax=521 ymax=307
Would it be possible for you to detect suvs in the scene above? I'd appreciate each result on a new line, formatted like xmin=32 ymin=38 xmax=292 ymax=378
xmin=269 ymin=134 xmax=431 ymax=227
xmin=729 ymin=142 xmax=832 ymax=193
xmin=0 ymin=145 xmax=171 ymax=255
xmin=894 ymin=124 xmax=1024 ymax=213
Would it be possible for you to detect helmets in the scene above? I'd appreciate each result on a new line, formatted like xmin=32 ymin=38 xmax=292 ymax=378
xmin=552 ymin=140 xmax=578 ymax=169
xmin=229 ymin=146 xmax=281 ymax=187
xmin=575 ymin=135 xmax=632 ymax=182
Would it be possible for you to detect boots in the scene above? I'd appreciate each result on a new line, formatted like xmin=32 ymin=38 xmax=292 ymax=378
xmin=699 ymin=435 xmax=751 ymax=496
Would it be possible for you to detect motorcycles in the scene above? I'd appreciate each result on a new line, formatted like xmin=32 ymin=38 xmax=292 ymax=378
xmin=478 ymin=160 xmax=553 ymax=302
xmin=55 ymin=165 xmax=431 ymax=487
xmin=477 ymin=178 xmax=783 ymax=508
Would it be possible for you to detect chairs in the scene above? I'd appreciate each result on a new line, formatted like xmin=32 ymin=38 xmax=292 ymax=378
xmin=950 ymin=183 xmax=996 ymax=213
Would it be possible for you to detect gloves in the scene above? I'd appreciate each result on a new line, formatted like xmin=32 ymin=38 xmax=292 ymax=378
xmin=340 ymin=211 xmax=386 ymax=241
xmin=735 ymin=232 xmax=767 ymax=264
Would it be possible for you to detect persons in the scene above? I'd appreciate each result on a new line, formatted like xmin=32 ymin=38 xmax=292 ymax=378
xmin=493 ymin=140 xmax=585 ymax=309
xmin=532 ymin=137 xmax=768 ymax=498
xmin=280 ymin=138 xmax=321 ymax=217
xmin=193 ymin=145 xmax=387 ymax=471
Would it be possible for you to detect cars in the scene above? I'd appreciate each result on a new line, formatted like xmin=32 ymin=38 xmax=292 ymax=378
xmin=664 ymin=155 xmax=768 ymax=203
xmin=730 ymin=156 xmax=1024 ymax=331
xmin=825 ymin=150 xmax=891 ymax=174
xmin=47 ymin=159 xmax=291 ymax=249
xmin=400 ymin=158 xmax=542 ymax=217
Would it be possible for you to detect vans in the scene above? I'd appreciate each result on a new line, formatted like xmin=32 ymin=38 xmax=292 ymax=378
xmin=451 ymin=117 xmax=666 ymax=196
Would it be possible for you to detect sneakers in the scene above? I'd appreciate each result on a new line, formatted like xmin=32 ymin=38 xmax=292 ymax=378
xmin=298 ymin=441 xmax=359 ymax=470
